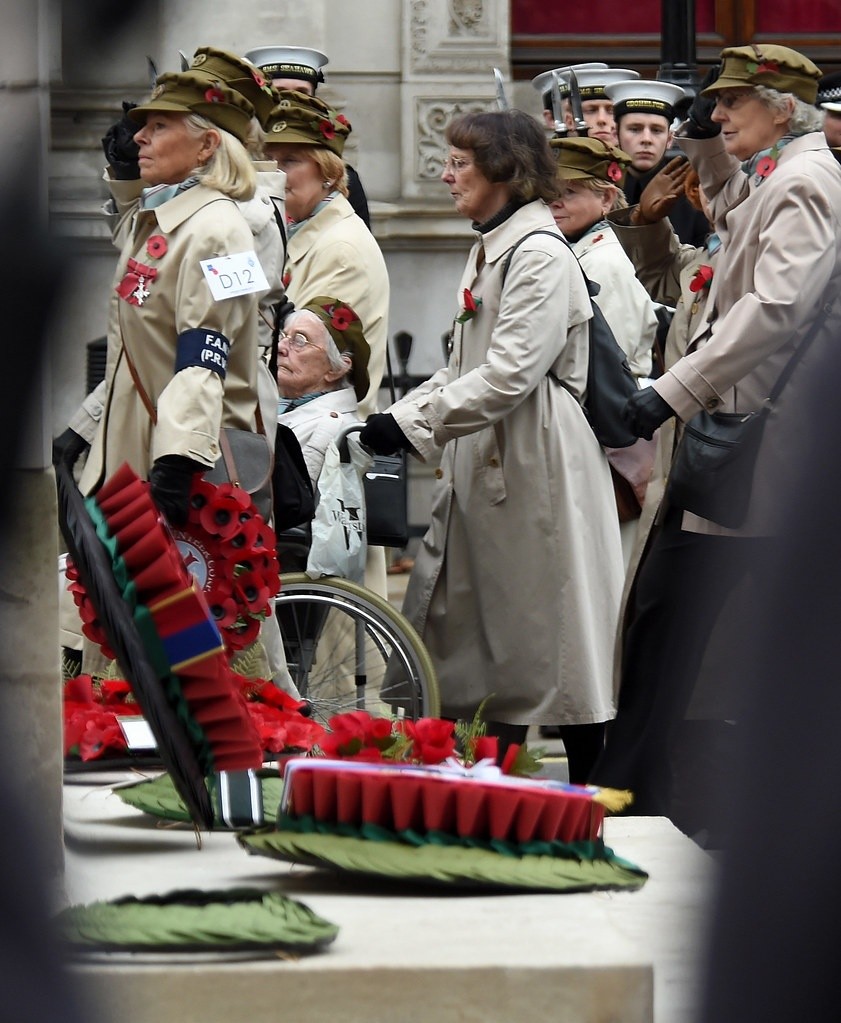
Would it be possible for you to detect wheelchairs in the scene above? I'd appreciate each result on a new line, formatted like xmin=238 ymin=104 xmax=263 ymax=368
xmin=258 ymin=422 xmax=441 ymax=768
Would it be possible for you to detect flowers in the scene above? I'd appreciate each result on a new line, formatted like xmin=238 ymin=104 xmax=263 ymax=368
xmin=747 ymin=61 xmax=778 ymax=73
xmin=324 ymin=300 xmax=352 ymax=330
xmin=146 ymin=235 xmax=168 ymax=260
xmin=690 ymin=264 xmax=713 ymax=303
xmin=329 ymin=112 xmax=348 ymax=127
xmin=608 ymin=162 xmax=625 ymax=181
xmin=252 ymin=73 xmax=273 ymax=96
xmin=310 ymin=121 xmax=336 ymax=140
xmin=59 ymin=476 xmax=649 ymax=884
xmin=282 ymin=267 xmax=292 ymax=291
xmin=455 ymin=288 xmax=483 ymax=325
xmin=756 ymin=143 xmax=779 ymax=178
xmin=206 ymin=89 xmax=224 ymax=102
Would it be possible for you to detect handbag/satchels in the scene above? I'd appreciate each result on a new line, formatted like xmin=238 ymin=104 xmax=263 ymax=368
xmin=648 ymin=307 xmax=673 ymax=380
xmin=304 ymin=437 xmax=367 ymax=584
xmin=666 ymin=407 xmax=771 ymax=530
xmin=272 ymin=422 xmax=316 ymax=533
xmin=199 ymin=426 xmax=272 ymax=524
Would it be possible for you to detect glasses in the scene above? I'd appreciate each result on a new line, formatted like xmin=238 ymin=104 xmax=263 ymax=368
xmin=442 ymin=157 xmax=475 ymax=175
xmin=278 ymin=329 xmax=326 ymax=353
xmin=713 ymin=91 xmax=754 ymax=108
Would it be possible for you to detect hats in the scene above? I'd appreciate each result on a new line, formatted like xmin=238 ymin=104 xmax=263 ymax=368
xmin=301 ymin=296 xmax=371 ymax=403
xmin=549 ymin=137 xmax=632 ymax=191
xmin=187 ymin=46 xmax=353 ymax=159
xmin=126 ymin=71 xmax=256 ymax=144
xmin=815 ymin=71 xmax=841 ymax=112
xmin=531 ymin=62 xmax=686 ymax=125
xmin=699 ymin=43 xmax=823 ymax=106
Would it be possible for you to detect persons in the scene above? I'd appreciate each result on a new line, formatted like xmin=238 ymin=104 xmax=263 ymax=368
xmin=535 ymin=43 xmax=841 ymax=872
xmin=54 ymin=43 xmax=391 ymax=735
xmin=361 ymin=109 xmax=625 ymax=788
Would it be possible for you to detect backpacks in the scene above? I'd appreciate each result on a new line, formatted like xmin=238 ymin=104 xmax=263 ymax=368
xmin=501 ymin=230 xmax=642 ymax=449
xmin=361 ymin=453 xmax=410 ymax=548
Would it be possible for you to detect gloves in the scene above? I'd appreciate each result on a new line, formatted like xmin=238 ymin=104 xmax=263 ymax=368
xmin=360 ymin=413 xmax=407 ymax=456
xmin=629 ymin=155 xmax=693 ymax=227
xmin=621 ymin=385 xmax=675 ymax=441
xmin=150 ymin=454 xmax=196 ymax=527
xmin=687 ymin=64 xmax=721 ymax=131
xmin=51 ymin=426 xmax=89 ymax=471
xmin=102 ymin=100 xmax=147 ymax=179
xmin=267 ymin=294 xmax=295 ymax=384
